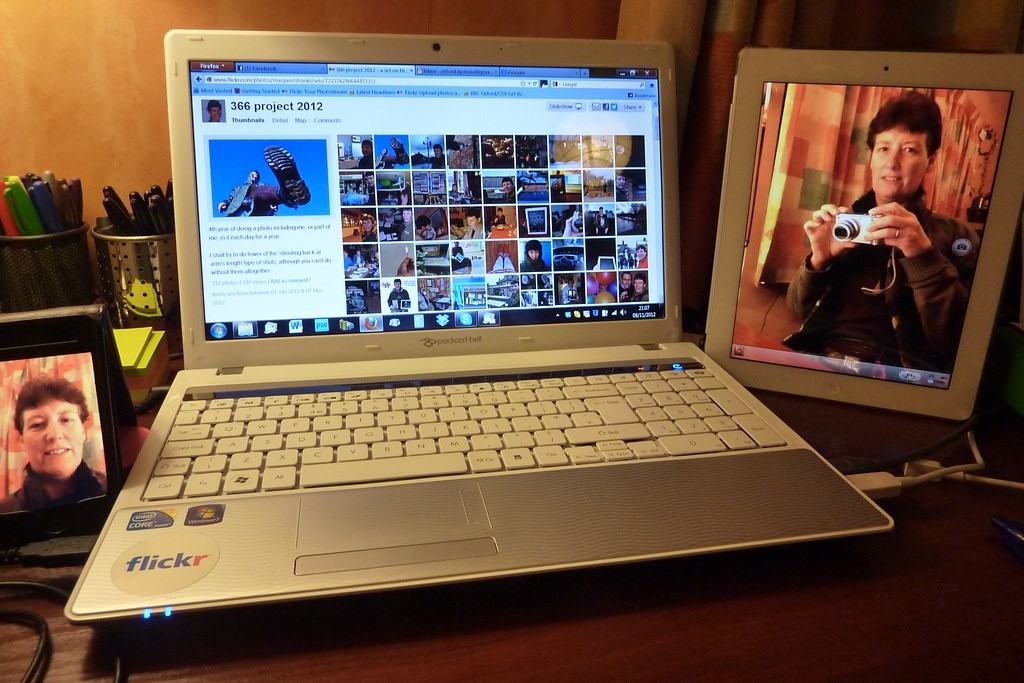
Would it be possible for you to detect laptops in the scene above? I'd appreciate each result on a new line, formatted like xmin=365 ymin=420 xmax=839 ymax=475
xmin=64 ymin=26 xmax=895 ymax=628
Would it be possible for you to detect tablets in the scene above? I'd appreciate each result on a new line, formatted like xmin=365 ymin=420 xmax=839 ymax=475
xmin=704 ymin=47 xmax=1024 ymax=421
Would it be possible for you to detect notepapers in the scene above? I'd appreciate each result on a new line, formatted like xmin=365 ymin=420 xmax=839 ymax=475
xmin=109 ymin=325 xmax=172 ymax=411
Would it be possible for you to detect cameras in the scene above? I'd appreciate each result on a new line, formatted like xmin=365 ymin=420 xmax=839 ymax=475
xmin=833 ymin=213 xmax=884 ymax=245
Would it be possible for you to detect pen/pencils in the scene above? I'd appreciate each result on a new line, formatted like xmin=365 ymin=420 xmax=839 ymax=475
xmin=0 ymin=170 xmax=97 ymax=313
xmin=95 ymin=175 xmax=174 ymax=235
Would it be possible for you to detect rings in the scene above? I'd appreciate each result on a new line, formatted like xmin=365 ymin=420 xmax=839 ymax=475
xmin=895 ymin=229 xmax=900 ymax=238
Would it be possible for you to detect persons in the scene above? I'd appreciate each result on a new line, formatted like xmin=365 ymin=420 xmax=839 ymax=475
xmin=616 ymin=174 xmax=629 ymax=201
xmin=206 ymin=100 xmax=222 ymax=122
xmin=217 ymin=146 xmax=311 ymax=217
xmin=0 ymin=375 xmax=107 ymax=513
xmin=338 ymin=139 xmax=582 ymax=313
xmin=619 ymin=246 xmax=650 ymax=302
xmin=595 ymin=207 xmax=608 ymax=236
xmin=784 ymin=91 xmax=978 ymax=374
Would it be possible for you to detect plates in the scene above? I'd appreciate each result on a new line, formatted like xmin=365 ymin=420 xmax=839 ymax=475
xmin=357 ymin=268 xmax=368 ymax=273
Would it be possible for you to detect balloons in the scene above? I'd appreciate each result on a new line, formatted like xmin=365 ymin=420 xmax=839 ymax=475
xmin=586 ymin=272 xmax=617 ymax=304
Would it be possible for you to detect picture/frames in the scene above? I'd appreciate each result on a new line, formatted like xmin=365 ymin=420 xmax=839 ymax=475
xmin=0 ymin=338 xmax=121 ymax=536
